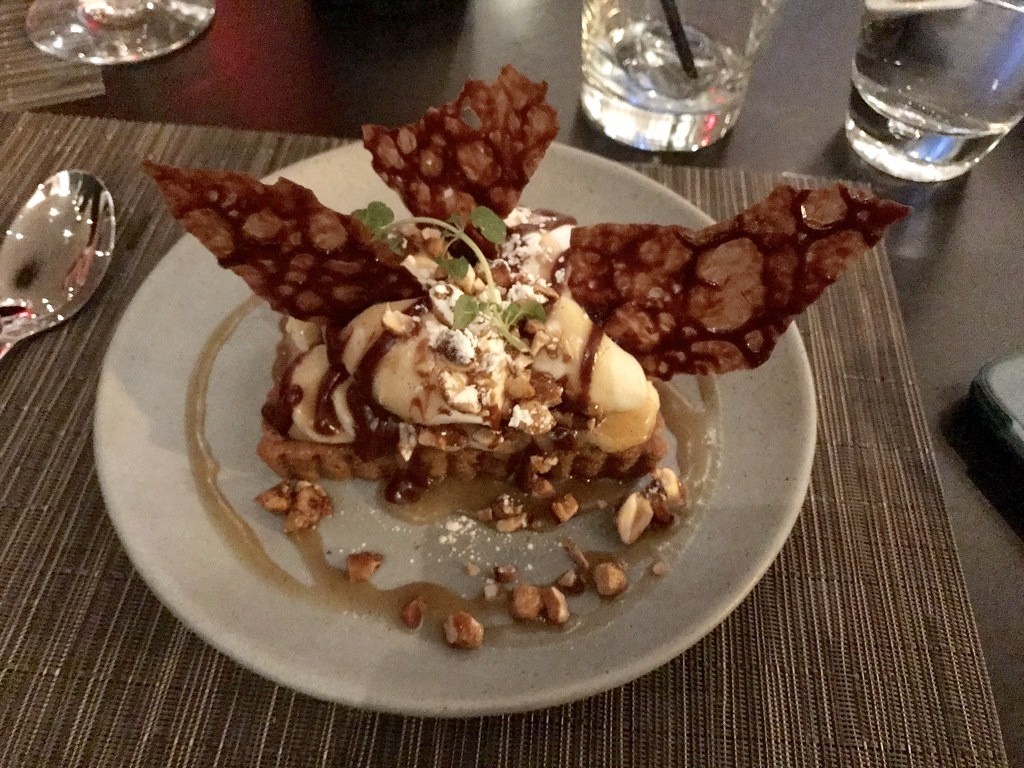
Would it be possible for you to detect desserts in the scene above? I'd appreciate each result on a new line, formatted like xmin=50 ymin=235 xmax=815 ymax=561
xmin=139 ymin=62 xmax=914 ymax=648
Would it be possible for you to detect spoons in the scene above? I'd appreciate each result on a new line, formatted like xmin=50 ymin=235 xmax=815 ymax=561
xmin=0 ymin=166 xmax=116 ymax=361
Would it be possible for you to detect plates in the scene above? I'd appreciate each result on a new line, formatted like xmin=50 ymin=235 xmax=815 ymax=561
xmin=91 ymin=137 xmax=819 ymax=721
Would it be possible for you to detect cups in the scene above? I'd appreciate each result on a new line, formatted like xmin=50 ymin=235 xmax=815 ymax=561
xmin=577 ymin=0 xmax=781 ymax=154
xmin=844 ymin=0 xmax=1024 ymax=185
